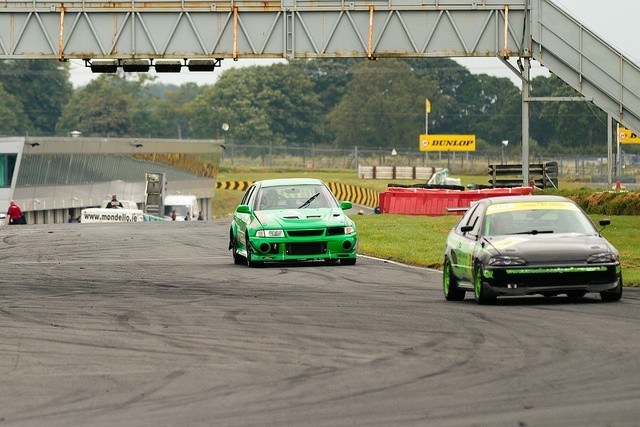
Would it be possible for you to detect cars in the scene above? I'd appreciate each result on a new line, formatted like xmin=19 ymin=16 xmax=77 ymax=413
xmin=443 ymin=195 xmax=623 ymax=305
xmin=228 ymin=177 xmax=358 ymax=268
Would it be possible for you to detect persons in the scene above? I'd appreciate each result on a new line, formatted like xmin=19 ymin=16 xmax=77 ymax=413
xmin=197 ymin=211 xmax=204 ymax=221
xmin=171 ymin=209 xmax=177 ymax=221
xmin=8 ymin=200 xmax=23 ymax=225
xmin=184 ymin=212 xmax=192 ymax=220
xmin=106 ymin=194 xmax=123 ymax=208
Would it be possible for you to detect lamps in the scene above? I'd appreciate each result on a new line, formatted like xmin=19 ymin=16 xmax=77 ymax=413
xmin=82 ymin=58 xmax=225 ymax=73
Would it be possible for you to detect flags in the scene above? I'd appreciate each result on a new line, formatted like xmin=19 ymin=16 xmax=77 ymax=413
xmin=427 ymin=99 xmax=431 ymax=113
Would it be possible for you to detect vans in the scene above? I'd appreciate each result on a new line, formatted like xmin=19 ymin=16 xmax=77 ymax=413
xmin=102 ymin=200 xmax=138 ymax=210
xmin=163 ymin=194 xmax=200 ymax=223
